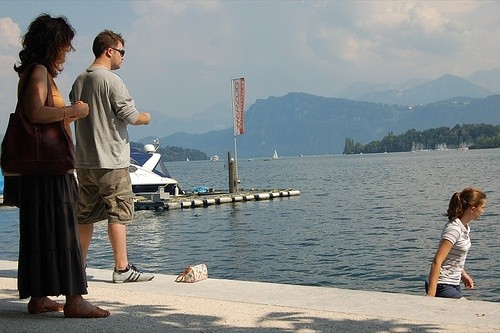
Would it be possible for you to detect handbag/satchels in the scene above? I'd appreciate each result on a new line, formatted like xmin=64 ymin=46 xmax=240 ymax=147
xmin=175 ymin=263 xmax=208 ymax=283
xmin=0 ymin=63 xmax=76 ymax=175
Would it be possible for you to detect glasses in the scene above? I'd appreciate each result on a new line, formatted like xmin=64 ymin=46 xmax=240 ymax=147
xmin=110 ymin=46 xmax=125 ymax=57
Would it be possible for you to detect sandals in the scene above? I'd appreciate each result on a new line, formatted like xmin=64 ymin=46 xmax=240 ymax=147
xmin=62 ymin=304 xmax=111 ymax=318
xmin=26 ymin=301 xmax=62 ymax=312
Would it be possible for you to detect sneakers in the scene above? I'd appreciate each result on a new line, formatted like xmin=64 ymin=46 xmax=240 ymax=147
xmin=112 ymin=264 xmax=154 ymax=283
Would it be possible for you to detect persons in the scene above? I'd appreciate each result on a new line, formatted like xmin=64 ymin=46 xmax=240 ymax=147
xmin=425 ymin=187 xmax=487 ymax=301
xmin=0 ymin=13 xmax=111 ymax=318
xmin=69 ymin=29 xmax=155 ymax=283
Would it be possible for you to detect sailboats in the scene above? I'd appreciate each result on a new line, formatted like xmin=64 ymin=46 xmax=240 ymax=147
xmin=272 ymin=149 xmax=279 ymax=160
xmin=412 ymin=142 xmax=468 ymax=152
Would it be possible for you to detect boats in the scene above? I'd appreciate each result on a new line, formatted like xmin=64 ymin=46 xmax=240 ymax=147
xmin=130 ymin=139 xmax=184 ymax=195
xmin=210 ymin=155 xmax=219 ymax=161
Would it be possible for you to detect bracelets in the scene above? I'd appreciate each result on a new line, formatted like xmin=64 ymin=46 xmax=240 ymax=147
xmin=63 ymin=106 xmax=67 ymax=120
